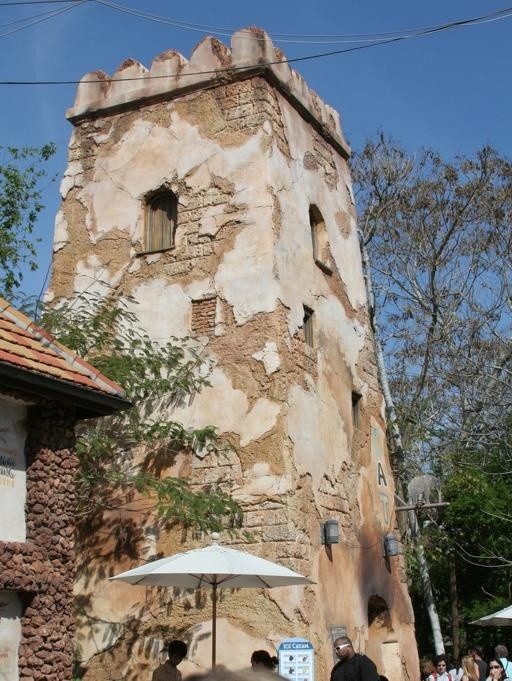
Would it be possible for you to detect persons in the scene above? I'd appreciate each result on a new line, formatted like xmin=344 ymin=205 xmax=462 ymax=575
xmin=152 ymin=641 xmax=187 ymax=681
xmin=419 ymin=643 xmax=512 ymax=681
xmin=249 ymin=648 xmax=279 ymax=674
xmin=329 ymin=636 xmax=380 ymax=681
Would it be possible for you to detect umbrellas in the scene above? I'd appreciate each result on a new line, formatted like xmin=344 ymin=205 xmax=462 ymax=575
xmin=108 ymin=531 xmax=320 ymax=671
xmin=467 ymin=605 xmax=512 ymax=628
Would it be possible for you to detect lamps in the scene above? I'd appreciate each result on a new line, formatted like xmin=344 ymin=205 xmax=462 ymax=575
xmin=380 ymin=533 xmax=400 ymax=558
xmin=321 ymin=519 xmax=340 ymax=545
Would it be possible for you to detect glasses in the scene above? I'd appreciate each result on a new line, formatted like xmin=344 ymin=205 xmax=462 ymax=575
xmin=489 ymin=667 xmax=498 ymax=670
xmin=335 ymin=644 xmax=349 ymax=651
